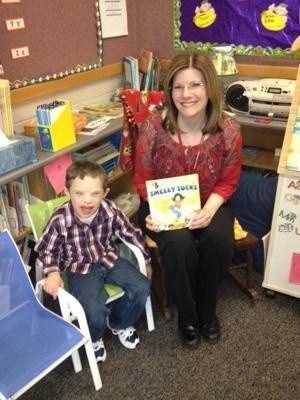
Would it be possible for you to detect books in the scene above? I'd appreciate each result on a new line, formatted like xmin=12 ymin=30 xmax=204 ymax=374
xmin=73 ymin=138 xmax=121 ymax=168
xmin=0 ymin=175 xmax=39 ymax=318
xmin=0 ymin=49 xmax=164 ymax=136
xmin=143 ymin=174 xmax=202 ymax=233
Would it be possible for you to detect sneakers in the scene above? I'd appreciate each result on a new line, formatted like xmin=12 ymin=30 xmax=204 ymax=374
xmin=92 ymin=338 xmax=107 ymax=363
xmin=107 ymin=316 xmax=139 ymax=350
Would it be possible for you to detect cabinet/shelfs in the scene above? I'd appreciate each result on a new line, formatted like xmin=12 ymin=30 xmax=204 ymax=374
xmin=1 ymin=89 xmax=151 ymax=240
xmin=242 ymin=123 xmax=285 ymax=172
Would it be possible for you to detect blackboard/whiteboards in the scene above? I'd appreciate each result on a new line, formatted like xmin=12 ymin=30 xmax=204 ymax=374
xmin=276 ymin=61 xmax=300 ymax=179
xmin=261 ymin=175 xmax=300 ymax=298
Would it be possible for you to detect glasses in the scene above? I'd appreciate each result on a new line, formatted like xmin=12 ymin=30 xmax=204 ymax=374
xmin=168 ymin=83 xmax=205 ymax=92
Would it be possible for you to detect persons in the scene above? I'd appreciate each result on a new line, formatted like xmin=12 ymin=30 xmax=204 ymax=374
xmin=37 ymin=160 xmax=154 ymax=366
xmin=136 ymin=51 xmax=243 ymax=351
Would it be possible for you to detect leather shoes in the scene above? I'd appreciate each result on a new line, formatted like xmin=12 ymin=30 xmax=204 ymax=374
xmin=177 ymin=317 xmax=201 ymax=348
xmin=201 ymin=309 xmax=221 ymax=343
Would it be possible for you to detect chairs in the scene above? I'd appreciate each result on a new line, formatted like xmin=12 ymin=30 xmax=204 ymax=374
xmin=120 ymin=90 xmax=260 ymax=321
xmin=26 ymin=196 xmax=155 ymax=373
xmin=1 ymin=229 xmax=102 ymax=400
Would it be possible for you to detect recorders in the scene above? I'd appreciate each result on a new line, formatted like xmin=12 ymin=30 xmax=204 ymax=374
xmin=225 ymin=78 xmax=296 ymax=121
